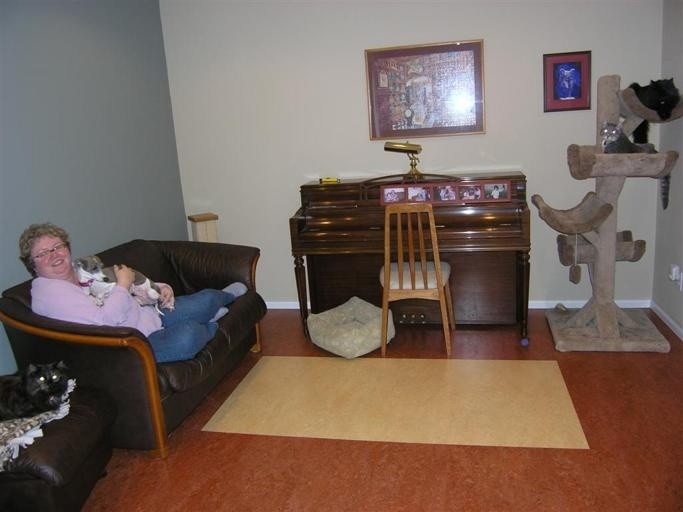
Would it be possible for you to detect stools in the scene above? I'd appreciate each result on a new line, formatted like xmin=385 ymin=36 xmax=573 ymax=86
xmin=308 ymin=296 xmax=395 ymax=359
xmin=0 ymin=387 xmax=119 ymax=512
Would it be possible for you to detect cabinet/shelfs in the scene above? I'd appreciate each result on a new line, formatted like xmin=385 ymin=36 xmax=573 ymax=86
xmin=289 ymin=171 xmax=531 ymax=338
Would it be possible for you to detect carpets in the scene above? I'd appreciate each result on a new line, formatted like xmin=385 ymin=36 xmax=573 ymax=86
xmin=201 ymin=355 xmax=589 ymax=449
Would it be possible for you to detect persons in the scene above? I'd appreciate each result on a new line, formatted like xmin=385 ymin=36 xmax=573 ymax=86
xmin=384 ymin=184 xmax=505 ymax=203
xmin=19 ymin=220 xmax=248 ymax=364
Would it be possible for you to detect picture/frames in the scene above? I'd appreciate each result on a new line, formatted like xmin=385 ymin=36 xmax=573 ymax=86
xmin=379 ymin=180 xmax=511 ymax=205
xmin=364 ymin=39 xmax=485 ymax=140
xmin=543 ymin=50 xmax=592 ymax=112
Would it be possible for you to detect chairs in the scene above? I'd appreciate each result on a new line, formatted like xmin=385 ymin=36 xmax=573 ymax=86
xmin=379 ymin=204 xmax=455 ymax=355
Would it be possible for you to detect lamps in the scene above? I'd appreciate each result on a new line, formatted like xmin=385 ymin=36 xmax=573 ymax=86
xmin=384 ymin=142 xmax=423 ymax=178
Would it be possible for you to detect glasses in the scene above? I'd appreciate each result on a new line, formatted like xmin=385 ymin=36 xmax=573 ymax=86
xmin=31 ymin=243 xmax=68 ymax=259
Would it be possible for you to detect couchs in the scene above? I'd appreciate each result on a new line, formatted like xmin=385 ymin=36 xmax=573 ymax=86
xmin=0 ymin=239 xmax=268 ymax=459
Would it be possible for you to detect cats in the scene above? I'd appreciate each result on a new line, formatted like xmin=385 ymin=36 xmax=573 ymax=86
xmin=0 ymin=359 xmax=70 ymax=421
xmin=600 ymin=120 xmax=671 ymax=210
xmin=628 ymin=78 xmax=681 ymax=144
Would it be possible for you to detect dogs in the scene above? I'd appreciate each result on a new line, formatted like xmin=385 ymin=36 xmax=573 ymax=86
xmin=70 ymin=255 xmax=161 ymax=307
